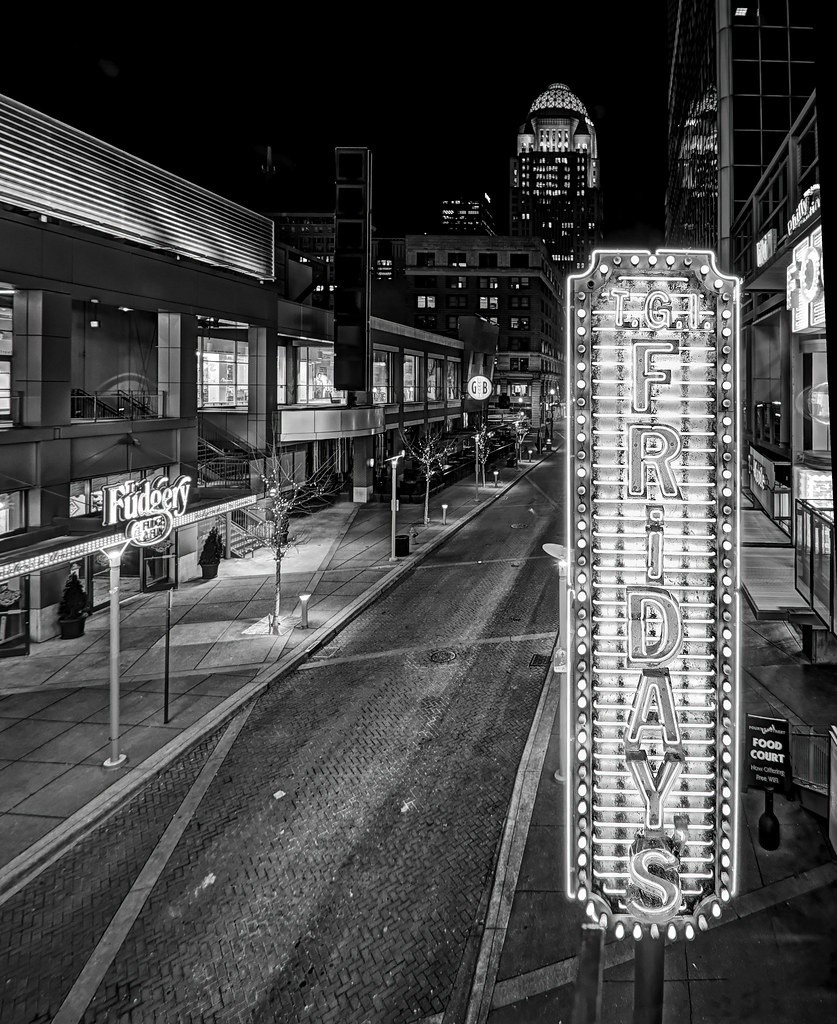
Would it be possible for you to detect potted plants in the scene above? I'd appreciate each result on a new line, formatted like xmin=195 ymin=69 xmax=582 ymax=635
xmin=197 ymin=526 xmax=223 ymax=578
xmin=58 ymin=571 xmax=89 ymax=639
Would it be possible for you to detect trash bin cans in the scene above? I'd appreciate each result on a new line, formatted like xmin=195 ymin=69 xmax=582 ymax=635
xmin=395 ymin=535 xmax=409 ymax=558
